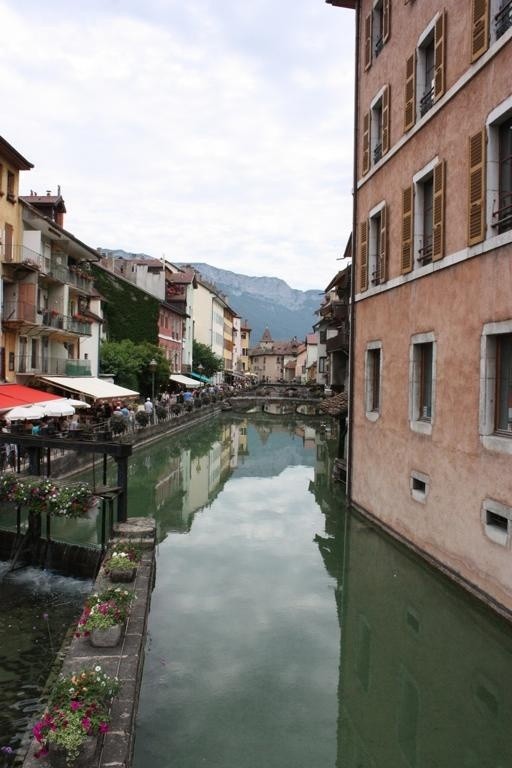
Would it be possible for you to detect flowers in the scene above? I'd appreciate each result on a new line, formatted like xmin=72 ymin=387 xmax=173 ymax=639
xmin=101 ymin=538 xmax=144 ymax=574
xmin=74 ymin=585 xmax=138 ymax=640
xmin=30 ymin=664 xmax=124 ymax=767
xmin=0 ymin=469 xmax=95 ymax=520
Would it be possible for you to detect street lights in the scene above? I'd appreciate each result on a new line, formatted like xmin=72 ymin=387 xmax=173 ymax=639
xmin=148 ymin=358 xmax=158 ymax=424
xmin=197 ymin=363 xmax=204 ymax=406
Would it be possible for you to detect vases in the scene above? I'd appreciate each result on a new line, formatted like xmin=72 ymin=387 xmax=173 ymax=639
xmin=87 ymin=625 xmax=123 ymax=649
xmin=110 ymin=570 xmax=135 ymax=582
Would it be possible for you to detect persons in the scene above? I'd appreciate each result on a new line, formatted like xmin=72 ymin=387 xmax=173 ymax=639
xmin=93 ymin=378 xmax=256 ymax=427
xmin=3 ymin=412 xmax=82 ymax=437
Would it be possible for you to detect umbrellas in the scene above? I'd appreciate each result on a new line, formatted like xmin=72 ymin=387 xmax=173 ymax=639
xmin=2 ymin=396 xmax=92 ymax=422
